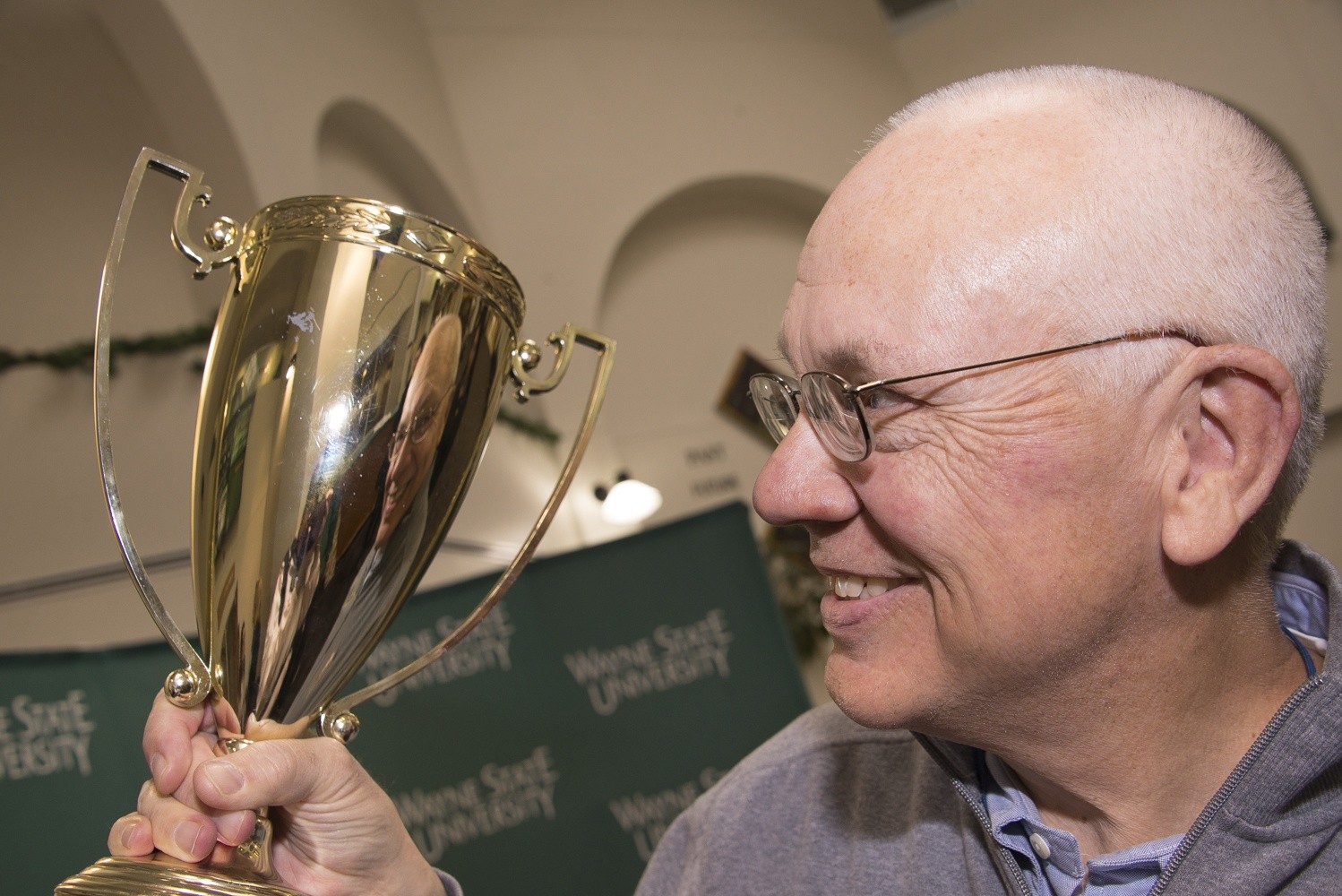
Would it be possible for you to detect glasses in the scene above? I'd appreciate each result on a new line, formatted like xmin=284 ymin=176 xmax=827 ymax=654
xmin=747 ymin=330 xmax=1210 ymax=463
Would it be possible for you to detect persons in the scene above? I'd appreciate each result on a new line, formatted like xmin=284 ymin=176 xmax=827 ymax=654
xmin=100 ymin=56 xmax=1339 ymax=896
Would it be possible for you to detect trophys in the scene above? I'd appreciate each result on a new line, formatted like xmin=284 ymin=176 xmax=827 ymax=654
xmin=47 ymin=145 xmax=621 ymax=896
